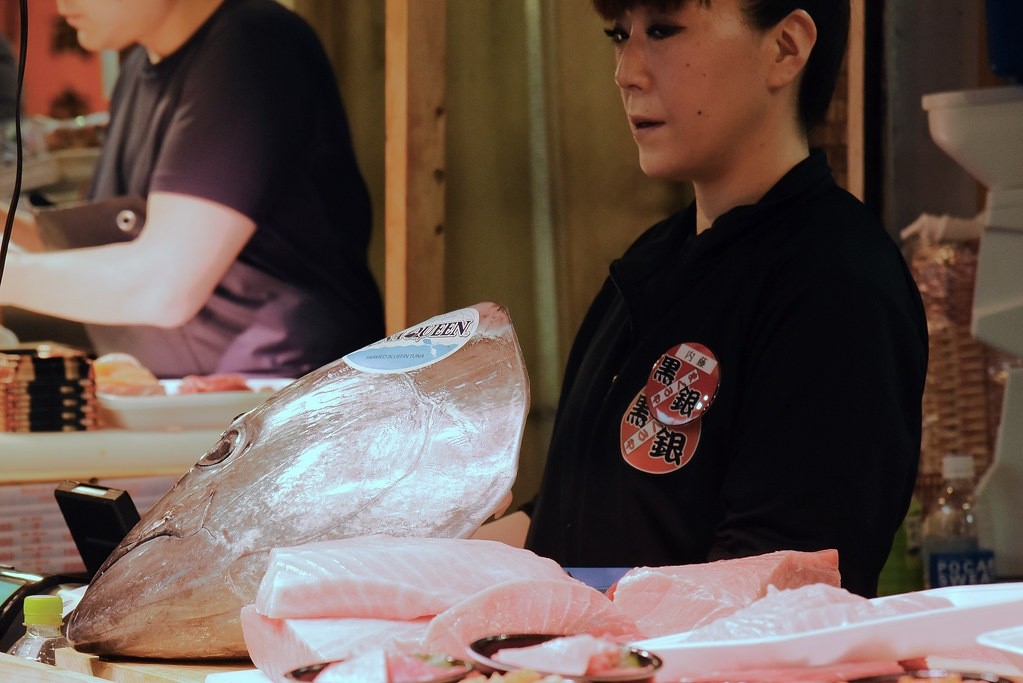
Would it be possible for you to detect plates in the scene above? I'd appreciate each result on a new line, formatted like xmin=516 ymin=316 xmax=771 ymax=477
xmin=97 ymin=378 xmax=301 ymax=428
xmin=633 ymin=581 xmax=1022 ymax=679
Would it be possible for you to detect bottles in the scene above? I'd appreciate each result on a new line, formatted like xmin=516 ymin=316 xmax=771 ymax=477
xmin=6 ymin=595 xmax=68 ymax=667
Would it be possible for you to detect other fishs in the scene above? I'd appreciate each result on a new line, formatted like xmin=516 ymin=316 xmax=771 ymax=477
xmin=66 ymin=300 xmax=532 ymax=659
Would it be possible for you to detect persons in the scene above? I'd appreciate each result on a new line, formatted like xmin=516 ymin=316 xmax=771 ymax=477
xmin=0 ymin=0 xmax=390 ymax=380
xmin=465 ymin=1 xmax=929 ymax=599
